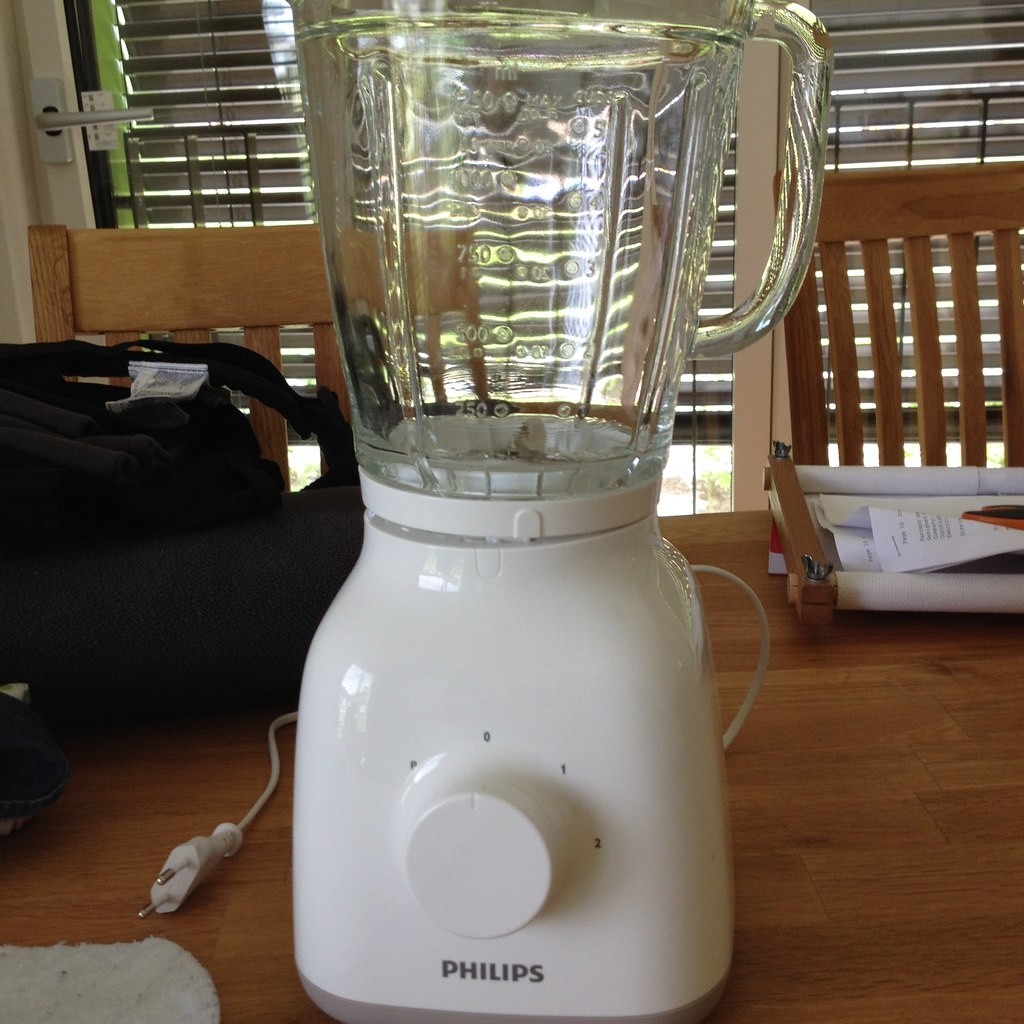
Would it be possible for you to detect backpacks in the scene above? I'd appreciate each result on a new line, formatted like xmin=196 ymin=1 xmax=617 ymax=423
xmin=0 ymin=339 xmax=359 ymax=538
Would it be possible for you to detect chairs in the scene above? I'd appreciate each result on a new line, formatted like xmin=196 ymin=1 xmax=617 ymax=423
xmin=774 ymin=160 xmax=1024 ymax=469
xmin=27 ymin=222 xmax=488 ymax=494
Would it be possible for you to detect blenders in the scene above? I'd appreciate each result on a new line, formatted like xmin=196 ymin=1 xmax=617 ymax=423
xmin=271 ymin=0 xmax=827 ymax=1024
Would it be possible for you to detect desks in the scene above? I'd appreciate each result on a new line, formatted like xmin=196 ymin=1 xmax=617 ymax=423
xmin=0 ymin=510 xmax=1024 ymax=1024
